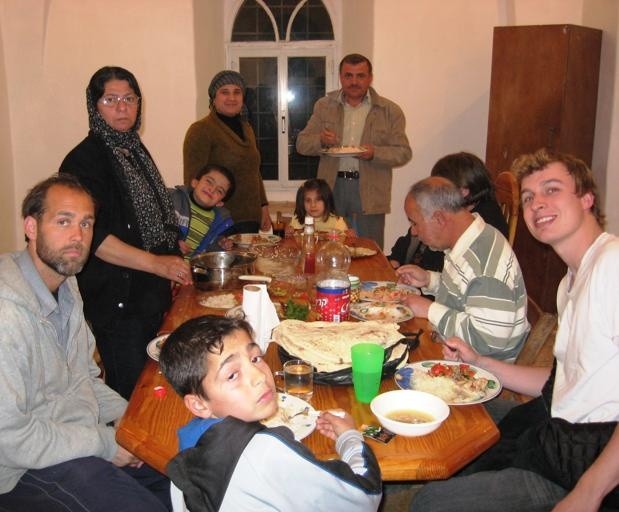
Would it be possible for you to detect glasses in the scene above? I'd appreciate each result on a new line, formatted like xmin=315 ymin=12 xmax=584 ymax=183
xmin=98 ymin=96 xmax=140 ymax=106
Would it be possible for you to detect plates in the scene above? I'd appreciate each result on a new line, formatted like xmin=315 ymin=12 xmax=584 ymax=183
xmin=195 ymin=290 xmax=242 ymax=311
xmin=343 ymin=245 xmax=377 ymax=258
xmin=350 ymin=281 xmax=422 ymax=323
xmin=147 ymin=335 xmax=171 ymax=364
xmin=227 ymin=231 xmax=281 ymax=245
xmin=321 ymin=147 xmax=367 ymax=158
xmin=262 ymin=393 xmax=318 ymax=442
xmin=395 ymin=360 xmax=502 ymax=406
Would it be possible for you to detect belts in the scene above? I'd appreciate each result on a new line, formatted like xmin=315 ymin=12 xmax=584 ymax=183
xmin=337 ymin=171 xmax=359 ymax=179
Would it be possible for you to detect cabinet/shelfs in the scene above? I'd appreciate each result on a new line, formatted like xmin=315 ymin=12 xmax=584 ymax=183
xmin=485 ymin=24 xmax=603 ymax=312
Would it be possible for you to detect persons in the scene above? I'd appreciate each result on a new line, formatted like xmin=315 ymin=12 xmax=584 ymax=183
xmin=0 ymin=172 xmax=173 ymax=512
xmin=388 ymin=150 xmax=510 ymax=302
xmin=394 ymin=178 xmax=531 ymax=364
xmin=165 ymin=162 xmax=239 ymax=288
xmin=183 ymin=70 xmax=271 ymax=235
xmin=58 ymin=66 xmax=198 ymax=401
xmin=295 ymin=54 xmax=411 ymax=251
xmin=290 ymin=178 xmax=351 ymax=236
xmin=442 ymin=147 xmax=619 ymax=511
xmin=158 ymin=306 xmax=568 ymax=512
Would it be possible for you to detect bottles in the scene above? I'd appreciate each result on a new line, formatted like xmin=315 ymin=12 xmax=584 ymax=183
xmin=301 ymin=215 xmax=360 ymax=323
xmin=285 ymin=225 xmax=295 ymax=239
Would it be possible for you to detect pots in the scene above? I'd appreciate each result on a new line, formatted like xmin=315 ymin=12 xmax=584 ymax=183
xmin=188 ymin=251 xmax=260 ymax=291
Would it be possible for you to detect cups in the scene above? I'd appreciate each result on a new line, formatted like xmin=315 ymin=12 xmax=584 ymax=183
xmin=274 ymin=359 xmax=314 ymax=402
xmin=268 ymin=275 xmax=312 ymax=296
xmin=351 ymin=343 xmax=385 ymax=403
xmin=272 ymin=221 xmax=285 ymax=238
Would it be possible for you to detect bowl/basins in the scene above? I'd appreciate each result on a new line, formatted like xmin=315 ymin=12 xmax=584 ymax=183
xmin=370 ymin=388 xmax=451 ymax=437
xmin=248 ymin=246 xmax=302 ymax=275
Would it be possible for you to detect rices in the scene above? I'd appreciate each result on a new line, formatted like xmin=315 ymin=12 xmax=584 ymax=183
xmin=201 ymin=292 xmax=237 ymax=310
xmin=327 ymin=144 xmax=363 ymax=153
xmin=409 ymin=369 xmax=481 ymax=404
xmin=257 ymin=405 xmax=294 ymax=431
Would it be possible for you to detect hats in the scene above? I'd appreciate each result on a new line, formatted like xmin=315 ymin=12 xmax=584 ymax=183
xmin=208 ymin=70 xmax=245 ymax=98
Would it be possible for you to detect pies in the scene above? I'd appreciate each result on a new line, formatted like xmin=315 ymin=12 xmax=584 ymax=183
xmin=273 ymin=318 xmax=410 ymax=375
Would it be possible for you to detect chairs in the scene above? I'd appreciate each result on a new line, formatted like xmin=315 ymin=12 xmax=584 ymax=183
xmin=495 ymin=170 xmax=519 ymax=248
xmin=500 ymin=294 xmax=557 ymax=399
